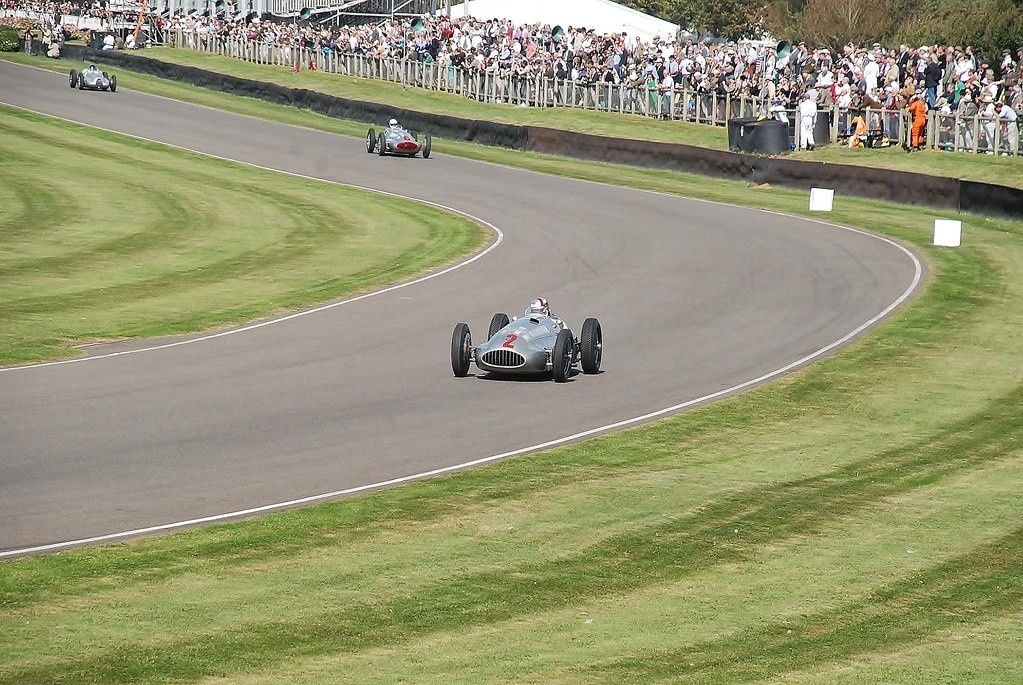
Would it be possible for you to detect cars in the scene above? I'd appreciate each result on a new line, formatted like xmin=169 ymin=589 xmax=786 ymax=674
xmin=451 ymin=313 xmax=603 ymax=382
xmin=69 ymin=68 xmax=116 ymax=92
xmin=365 ymin=128 xmax=432 ymax=158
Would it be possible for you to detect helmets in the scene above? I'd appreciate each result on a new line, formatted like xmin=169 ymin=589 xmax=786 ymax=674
xmin=531 ymin=297 xmax=548 ymax=315
xmin=389 ymin=119 xmax=398 ymax=127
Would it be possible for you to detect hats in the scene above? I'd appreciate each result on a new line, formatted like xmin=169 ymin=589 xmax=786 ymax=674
xmin=669 ymin=55 xmax=676 ymax=59
xmin=982 ymin=96 xmax=994 ymax=102
xmin=1003 ymin=49 xmax=1011 ymax=53
xmin=873 ymin=43 xmax=881 ymax=46
xmin=655 ymin=58 xmax=662 ymax=62
xmin=920 ymin=45 xmax=929 ymax=52
xmin=642 ymin=51 xmax=648 ymax=55
xmin=798 ymin=42 xmax=806 ymax=45
xmin=1016 ymin=47 xmax=1023 ymax=52
xmin=823 ymin=49 xmax=831 ymax=53
xmin=861 ymin=48 xmax=868 ymax=53
xmin=915 ymin=89 xmax=922 ymax=93
xmin=791 ymin=42 xmax=798 ymax=45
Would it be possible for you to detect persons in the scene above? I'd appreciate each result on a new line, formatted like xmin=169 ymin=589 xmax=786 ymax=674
xmin=1 ymin=0 xmax=1022 ymax=159
xmin=529 ymin=297 xmax=550 ymax=317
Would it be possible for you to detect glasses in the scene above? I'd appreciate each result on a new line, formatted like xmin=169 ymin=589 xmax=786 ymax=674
xmin=391 ymin=124 xmax=397 ymax=126
